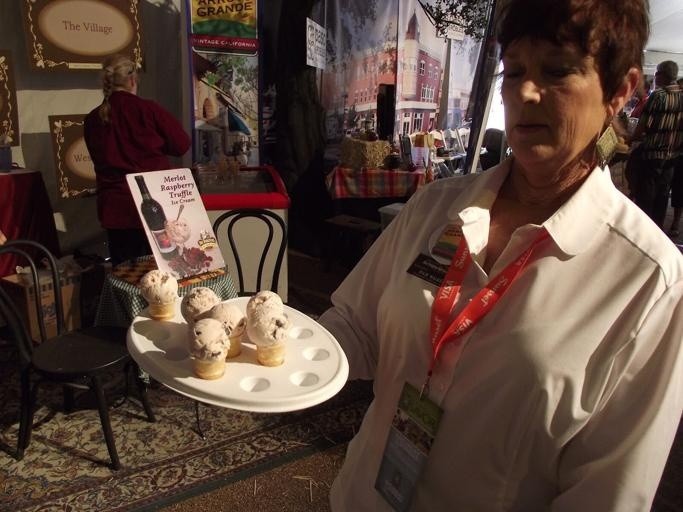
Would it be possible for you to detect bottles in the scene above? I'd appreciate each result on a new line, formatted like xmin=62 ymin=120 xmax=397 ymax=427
xmin=135 ymin=176 xmax=181 ymax=260
xmin=1 ymin=144 xmax=13 ymax=173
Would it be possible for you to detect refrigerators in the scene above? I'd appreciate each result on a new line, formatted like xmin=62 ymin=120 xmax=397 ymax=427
xmin=195 ymin=166 xmax=290 ymax=306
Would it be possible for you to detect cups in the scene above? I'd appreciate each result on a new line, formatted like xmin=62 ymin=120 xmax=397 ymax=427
xmin=165 ymin=220 xmax=192 ymax=246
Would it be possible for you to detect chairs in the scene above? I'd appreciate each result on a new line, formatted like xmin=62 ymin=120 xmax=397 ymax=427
xmin=1 ymin=238 xmax=156 ymax=472
xmin=211 ymin=208 xmax=288 ymax=297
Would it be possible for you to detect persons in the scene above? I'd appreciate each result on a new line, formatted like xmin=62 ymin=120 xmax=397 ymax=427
xmin=83 ymin=54 xmax=191 ymax=259
xmin=670 ymin=160 xmax=683 ymax=237
xmin=626 ymin=61 xmax=683 ymax=226
xmin=314 ymin=0 xmax=683 ymax=512
xmin=633 ymin=82 xmax=652 ymax=117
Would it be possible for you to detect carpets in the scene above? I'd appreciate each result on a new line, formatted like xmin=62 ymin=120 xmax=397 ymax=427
xmin=0 ymin=354 xmax=375 ymax=512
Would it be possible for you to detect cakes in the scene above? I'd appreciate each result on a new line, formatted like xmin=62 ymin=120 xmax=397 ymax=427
xmin=181 ymin=286 xmax=221 ymax=326
xmin=209 ymin=304 xmax=248 ymax=359
xmin=186 ymin=317 xmax=229 ymax=381
xmin=245 ymin=304 xmax=286 ymax=367
xmin=140 ymin=270 xmax=179 ymax=320
xmin=247 ymin=290 xmax=283 ymax=318
